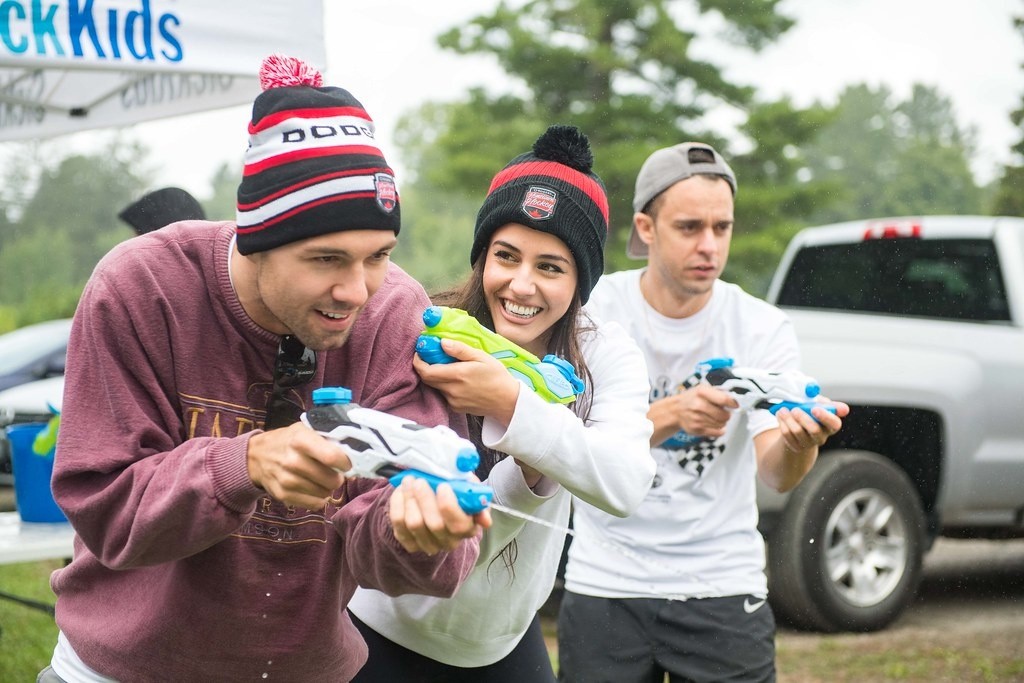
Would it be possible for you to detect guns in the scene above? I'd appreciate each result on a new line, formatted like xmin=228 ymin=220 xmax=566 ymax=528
xmin=291 ymin=383 xmax=496 ymax=520
xmin=702 ymin=356 xmax=838 ymax=430
xmin=417 ymin=302 xmax=586 ymax=412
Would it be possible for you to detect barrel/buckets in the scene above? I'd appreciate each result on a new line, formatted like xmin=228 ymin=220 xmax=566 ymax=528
xmin=6 ymin=422 xmax=70 ymax=524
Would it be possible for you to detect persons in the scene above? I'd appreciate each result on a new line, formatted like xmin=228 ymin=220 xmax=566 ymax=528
xmin=34 ymin=54 xmax=492 ymax=683
xmin=347 ymin=126 xmax=656 ymax=683
xmin=558 ymin=142 xmax=849 ymax=683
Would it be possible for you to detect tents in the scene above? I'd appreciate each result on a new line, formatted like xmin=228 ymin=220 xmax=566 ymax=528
xmin=0 ymin=0 xmax=327 ymax=140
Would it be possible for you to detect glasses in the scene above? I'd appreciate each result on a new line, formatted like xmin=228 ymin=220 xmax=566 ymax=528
xmin=264 ymin=333 xmax=317 ymax=432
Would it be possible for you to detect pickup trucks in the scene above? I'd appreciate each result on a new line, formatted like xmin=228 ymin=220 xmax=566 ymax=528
xmin=766 ymin=213 xmax=1024 ymax=634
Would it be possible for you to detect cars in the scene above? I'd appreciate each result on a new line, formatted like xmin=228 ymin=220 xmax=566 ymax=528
xmin=0 ymin=316 xmax=75 ymax=473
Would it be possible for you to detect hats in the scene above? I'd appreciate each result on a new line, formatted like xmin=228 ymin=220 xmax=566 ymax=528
xmin=471 ymin=126 xmax=610 ymax=307
xmin=625 ymin=142 xmax=737 ymax=260
xmin=235 ymin=52 xmax=401 ymax=257
